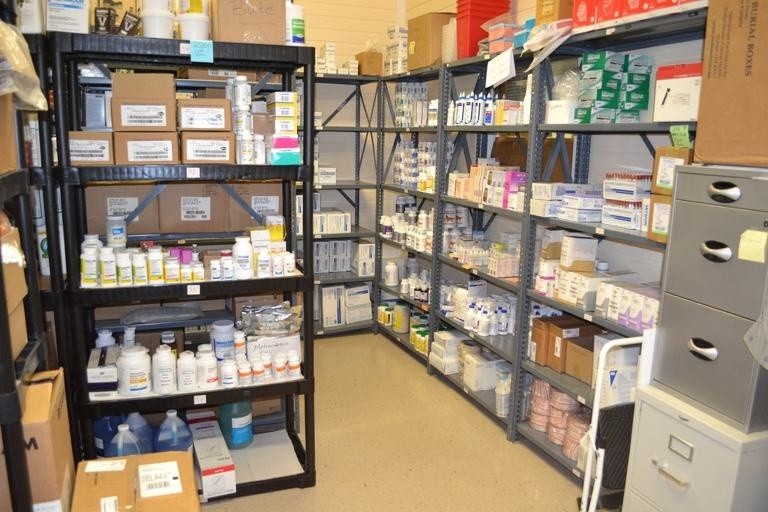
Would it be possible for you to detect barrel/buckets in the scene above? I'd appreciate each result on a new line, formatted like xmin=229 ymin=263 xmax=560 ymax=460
xmin=104 ymin=424 xmax=143 ymax=457
xmin=93 ymin=415 xmax=122 ymax=459
xmin=154 ymin=409 xmax=194 ymax=459
xmin=218 ymin=401 xmax=252 ymax=450
xmin=125 ymin=412 xmax=155 ymax=455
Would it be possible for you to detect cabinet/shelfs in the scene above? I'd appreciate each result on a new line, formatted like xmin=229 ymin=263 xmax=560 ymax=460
xmin=621 ymin=386 xmax=768 ymax=511
xmin=293 ymin=70 xmax=378 ymax=336
xmin=649 ymin=165 xmax=768 ymax=433
xmin=429 ymin=47 xmax=536 ymax=441
xmin=48 ymin=32 xmax=316 ymax=507
xmin=0 ymin=32 xmax=83 ymax=512
xmin=378 ymin=64 xmax=447 ymax=372
xmin=509 ymin=2 xmax=709 ymax=501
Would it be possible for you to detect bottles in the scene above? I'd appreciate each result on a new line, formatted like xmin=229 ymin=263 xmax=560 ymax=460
xmin=226 ymin=75 xmax=264 ymax=165
xmin=80 ymin=215 xmax=301 ymax=395
xmin=376 ymin=91 xmax=562 ymax=357
xmin=140 ymin=0 xmax=211 ymax=41
xmin=286 ymin=4 xmax=305 ymax=47
xmin=597 ymin=261 xmax=608 ymax=274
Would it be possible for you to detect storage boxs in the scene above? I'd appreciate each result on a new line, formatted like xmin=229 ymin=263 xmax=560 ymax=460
xmin=70 ymin=452 xmax=202 ymax=512
xmin=2 ymin=93 xmax=26 ymax=179
xmin=1 ymin=227 xmax=32 ymax=363
xmin=63 ymin=71 xmax=235 ymax=166
xmin=0 ymin=367 xmax=74 ymax=512
xmin=566 ymin=339 xmax=594 ymax=386
xmin=692 ymin=0 xmax=767 ymax=168
xmin=85 ymin=181 xmax=285 ymax=236
xmin=210 ymin=0 xmax=285 ymax=46
xmin=408 ymin=11 xmax=456 ymax=72
xmin=356 ymin=52 xmax=384 ymax=76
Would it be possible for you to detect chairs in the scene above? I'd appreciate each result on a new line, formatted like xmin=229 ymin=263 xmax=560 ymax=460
xmin=578 ymin=336 xmax=643 ymax=512
